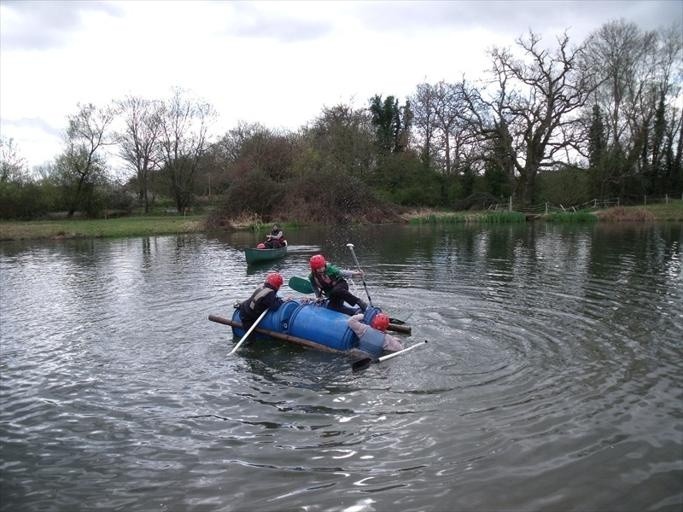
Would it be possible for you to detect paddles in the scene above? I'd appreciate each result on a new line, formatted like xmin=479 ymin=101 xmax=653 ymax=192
xmin=289 ymin=276 xmax=405 ymax=325
xmin=352 ymin=340 xmax=427 ymax=373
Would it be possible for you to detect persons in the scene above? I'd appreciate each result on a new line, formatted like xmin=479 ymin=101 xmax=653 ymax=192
xmin=346 ymin=312 xmax=405 ymax=362
xmin=306 ymin=254 xmax=369 ymax=318
xmin=236 ymin=272 xmax=291 ymax=348
xmin=263 ymin=223 xmax=284 ymax=248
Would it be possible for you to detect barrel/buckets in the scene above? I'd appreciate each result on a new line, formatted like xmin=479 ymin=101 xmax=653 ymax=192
xmin=288 ymin=304 xmax=360 ymax=350
xmin=314 ymin=297 xmax=378 ymax=325
xmin=230 ymin=299 xmax=299 ymax=340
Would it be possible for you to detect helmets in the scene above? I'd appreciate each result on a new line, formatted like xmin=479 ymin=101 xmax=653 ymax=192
xmin=308 ymin=253 xmax=326 ymax=271
xmin=263 ymin=272 xmax=284 ymax=291
xmin=371 ymin=312 xmax=391 ymax=331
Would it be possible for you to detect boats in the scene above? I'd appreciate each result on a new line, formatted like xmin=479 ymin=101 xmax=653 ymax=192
xmin=244 ymin=241 xmax=287 ymax=263
xmin=208 ymin=297 xmax=412 ymax=360
